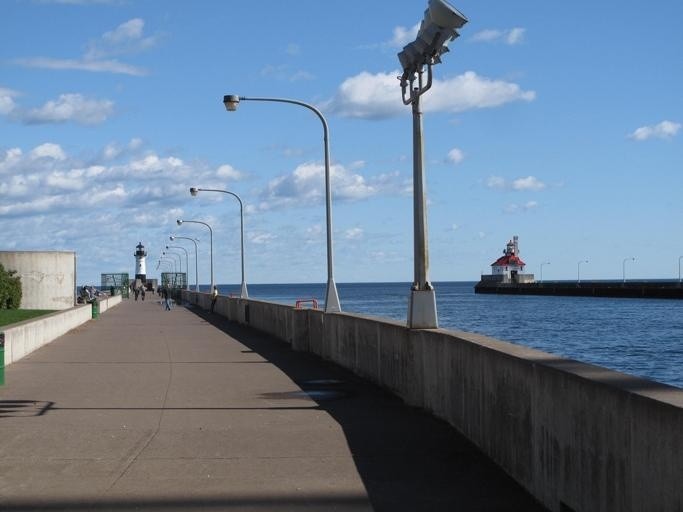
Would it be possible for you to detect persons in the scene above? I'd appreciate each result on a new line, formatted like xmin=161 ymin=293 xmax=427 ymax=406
xmin=209 ymin=285 xmax=218 ymax=313
xmin=110 ymin=285 xmax=115 ymax=296
xmin=129 ymin=282 xmax=146 ymax=301
xmin=152 ymin=285 xmax=155 ymax=295
xmin=77 ymin=285 xmax=101 ymax=304
xmin=157 ymin=283 xmax=171 ymax=311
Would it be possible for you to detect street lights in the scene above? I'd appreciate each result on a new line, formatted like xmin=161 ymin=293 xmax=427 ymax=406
xmin=577 ymin=259 xmax=589 ymax=283
xmin=169 ymin=236 xmax=198 ymax=294
xmin=397 ymin=1 xmax=469 ymax=329
xmin=622 ymin=257 xmax=636 ymax=283
xmin=189 ymin=187 xmax=248 ymax=299
xmin=539 ymin=262 xmax=551 ymax=285
xmin=155 ymin=246 xmax=190 ymax=291
xmin=222 ymin=95 xmax=344 ymax=315
xmin=176 ymin=220 xmax=215 ymax=293
